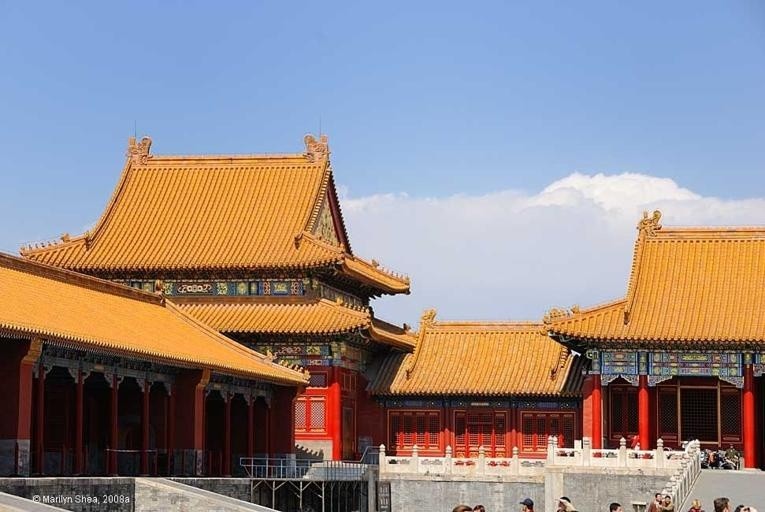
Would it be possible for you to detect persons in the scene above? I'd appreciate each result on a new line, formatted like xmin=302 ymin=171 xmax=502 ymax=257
xmin=520 ymin=499 xmax=533 ymax=512
xmin=452 ymin=505 xmax=472 ymax=512
xmin=688 ymin=499 xmax=705 ymax=512
xmin=610 ymin=503 xmax=622 ymax=512
xmin=735 ymin=505 xmax=750 ymax=512
xmin=663 ymin=496 xmax=674 ymax=512
xmin=557 ymin=497 xmax=579 ymax=512
xmin=634 ymin=434 xmax=740 ymax=470
xmin=714 ymin=498 xmax=731 ymax=512
xmin=473 ymin=505 xmax=485 ymax=512
xmin=648 ymin=493 xmax=664 ymax=512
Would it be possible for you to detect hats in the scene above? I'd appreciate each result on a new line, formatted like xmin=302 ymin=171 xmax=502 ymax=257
xmin=519 ymin=497 xmax=533 ymax=509
